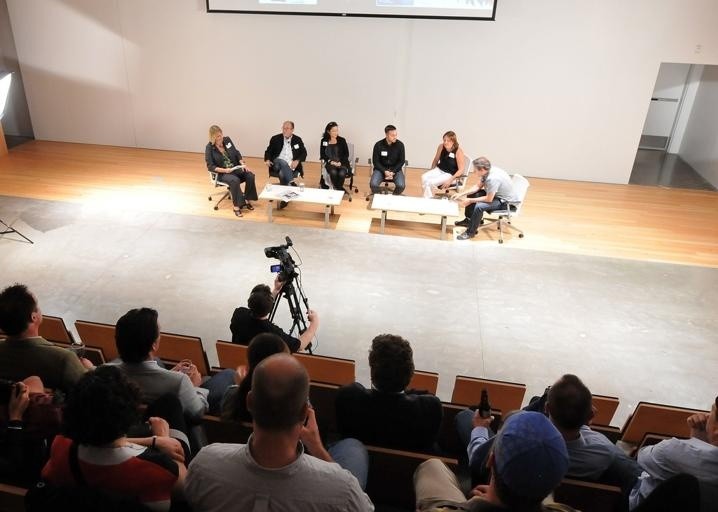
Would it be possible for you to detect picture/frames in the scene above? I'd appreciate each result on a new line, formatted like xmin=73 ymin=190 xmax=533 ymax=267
xmin=205 ymin=0 xmax=496 ymax=21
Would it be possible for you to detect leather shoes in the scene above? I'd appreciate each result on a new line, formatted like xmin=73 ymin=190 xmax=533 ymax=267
xmin=244 ymin=200 xmax=255 ymax=211
xmin=456 ymin=218 xmax=471 ymax=228
xmin=233 ymin=207 xmax=244 ymax=218
xmin=280 ymin=201 xmax=288 ymax=208
xmin=458 ymin=231 xmax=475 ymax=241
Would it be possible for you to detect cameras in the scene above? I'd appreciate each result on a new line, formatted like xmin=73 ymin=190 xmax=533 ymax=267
xmin=265 ymin=236 xmax=304 ymax=281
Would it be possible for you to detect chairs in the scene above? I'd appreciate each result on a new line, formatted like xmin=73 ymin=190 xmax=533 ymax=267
xmin=438 ymin=152 xmax=472 ymax=200
xmin=266 ymin=158 xmax=305 ymax=191
xmin=319 ymin=142 xmax=360 ymax=203
xmin=203 ymin=167 xmax=246 ymax=211
xmin=369 ymin=136 xmax=408 ymax=196
xmin=482 ymin=170 xmax=530 ymax=245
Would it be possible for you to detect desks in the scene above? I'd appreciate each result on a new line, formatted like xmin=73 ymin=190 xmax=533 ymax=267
xmin=367 ymin=193 xmax=460 ymax=238
xmin=255 ymin=183 xmax=344 ymax=227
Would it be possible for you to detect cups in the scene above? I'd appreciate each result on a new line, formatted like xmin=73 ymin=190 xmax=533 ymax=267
xmin=72 ymin=341 xmax=85 ymax=358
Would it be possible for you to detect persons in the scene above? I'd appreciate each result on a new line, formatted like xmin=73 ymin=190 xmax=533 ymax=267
xmin=229 ymin=275 xmax=319 ymax=353
xmin=370 ymin=124 xmax=406 ymax=196
xmin=209 ymin=333 xmax=291 ymax=420
xmin=637 ymin=397 xmax=718 ymax=512
xmin=1 ymin=285 xmax=208 ymax=511
xmin=420 ymin=131 xmax=466 ymax=200
xmin=450 ymin=156 xmax=520 ymax=242
xmin=334 ymin=334 xmax=442 ymax=454
xmin=317 ymin=122 xmax=351 ymax=193
xmin=175 ymin=353 xmax=376 ymax=512
xmin=410 ymin=411 xmax=582 ymax=512
xmin=467 ymin=374 xmax=642 ymax=485
xmin=205 ymin=125 xmax=258 ymax=218
xmin=263 ymin=122 xmax=307 ymax=211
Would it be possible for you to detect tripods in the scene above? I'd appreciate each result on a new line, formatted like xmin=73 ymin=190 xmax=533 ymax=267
xmin=266 ymin=281 xmax=314 ymax=356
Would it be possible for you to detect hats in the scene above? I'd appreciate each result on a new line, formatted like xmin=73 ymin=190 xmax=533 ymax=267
xmin=496 ymin=410 xmax=572 ymax=499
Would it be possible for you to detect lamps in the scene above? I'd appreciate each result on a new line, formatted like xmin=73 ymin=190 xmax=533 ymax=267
xmin=0 ymin=68 xmax=34 ymax=244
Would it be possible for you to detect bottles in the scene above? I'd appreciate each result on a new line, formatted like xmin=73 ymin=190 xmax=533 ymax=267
xmin=299 ymin=176 xmax=305 ymax=192
xmin=479 ymin=387 xmax=491 ymax=419
